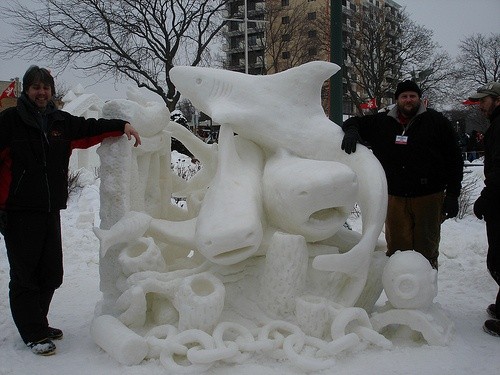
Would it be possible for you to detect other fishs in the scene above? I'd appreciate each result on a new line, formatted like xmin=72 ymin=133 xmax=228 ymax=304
xmin=93 ymin=211 xmax=151 ymax=258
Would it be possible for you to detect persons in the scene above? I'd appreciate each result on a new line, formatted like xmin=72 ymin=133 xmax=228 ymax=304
xmin=468 ymin=82 xmax=500 ymax=338
xmin=341 ymin=80 xmax=464 ymax=271
xmin=0 ymin=65 xmax=142 ymax=356
xmin=461 ymin=130 xmax=486 ymax=161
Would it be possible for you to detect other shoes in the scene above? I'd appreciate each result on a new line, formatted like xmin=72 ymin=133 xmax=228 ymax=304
xmin=31 ymin=337 xmax=57 ymax=356
xmin=47 ymin=327 xmax=63 ymax=339
xmin=484 ymin=320 xmax=500 ymax=337
xmin=487 ymin=304 xmax=500 ymax=319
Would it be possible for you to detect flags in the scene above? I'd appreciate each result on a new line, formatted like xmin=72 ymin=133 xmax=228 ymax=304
xmin=463 ymin=100 xmax=480 ymax=104
xmin=359 ymin=97 xmax=377 ymax=108
xmin=0 ymin=81 xmax=17 ymax=98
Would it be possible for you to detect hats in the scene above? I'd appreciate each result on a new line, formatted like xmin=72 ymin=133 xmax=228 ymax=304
xmin=469 ymin=83 xmax=500 ymax=101
xmin=395 ymin=80 xmax=422 ymax=100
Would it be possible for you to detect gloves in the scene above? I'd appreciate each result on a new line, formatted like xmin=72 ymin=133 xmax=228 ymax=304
xmin=474 ymin=196 xmax=484 ymax=220
xmin=443 ymin=196 xmax=459 ymax=218
xmin=341 ymin=128 xmax=358 ymax=154
